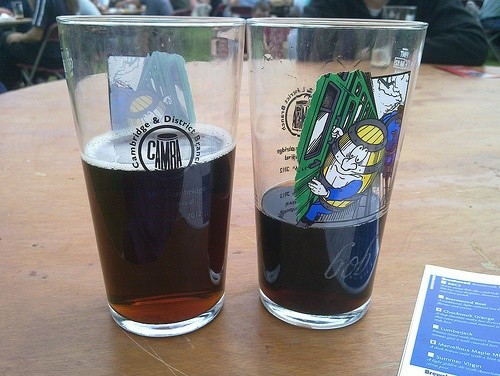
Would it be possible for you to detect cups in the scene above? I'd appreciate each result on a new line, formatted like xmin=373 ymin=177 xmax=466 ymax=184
xmin=381 ymin=6 xmax=416 ymax=21
xmin=56 ymin=15 xmax=246 ymax=337
xmin=11 ymin=1 xmax=26 ymax=19
xmin=246 ymin=18 xmax=429 ymax=330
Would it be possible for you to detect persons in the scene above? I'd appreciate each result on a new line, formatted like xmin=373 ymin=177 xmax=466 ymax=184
xmin=0 ymin=0 xmax=500 ymax=93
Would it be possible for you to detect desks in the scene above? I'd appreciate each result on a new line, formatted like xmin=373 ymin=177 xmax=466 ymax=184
xmin=0 ymin=59 xmax=500 ymax=376
